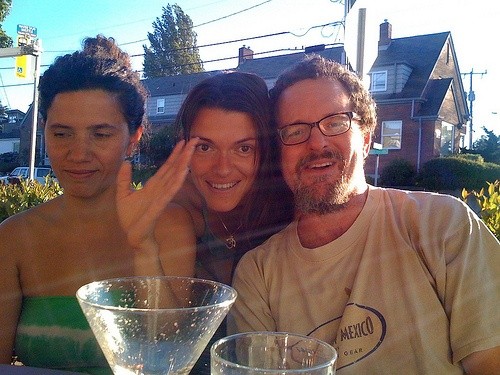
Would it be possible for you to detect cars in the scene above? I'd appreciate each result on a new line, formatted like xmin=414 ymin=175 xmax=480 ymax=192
xmin=1 ymin=167 xmax=51 ymax=187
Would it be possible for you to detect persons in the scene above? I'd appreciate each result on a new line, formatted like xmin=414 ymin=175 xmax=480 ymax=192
xmin=227 ymin=58 xmax=500 ymax=375
xmin=169 ymin=73 xmax=294 ymax=375
xmin=0 ymin=37 xmax=199 ymax=375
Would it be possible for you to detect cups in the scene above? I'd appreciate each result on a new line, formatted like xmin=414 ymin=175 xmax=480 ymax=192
xmin=75 ymin=276 xmax=238 ymax=375
xmin=210 ymin=330 xmax=339 ymax=375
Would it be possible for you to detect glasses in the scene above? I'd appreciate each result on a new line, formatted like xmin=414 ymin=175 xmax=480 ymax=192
xmin=275 ymin=111 xmax=363 ymax=145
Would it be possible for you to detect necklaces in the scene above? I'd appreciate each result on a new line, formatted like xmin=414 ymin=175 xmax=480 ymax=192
xmin=218 ymin=215 xmax=241 ymax=249
